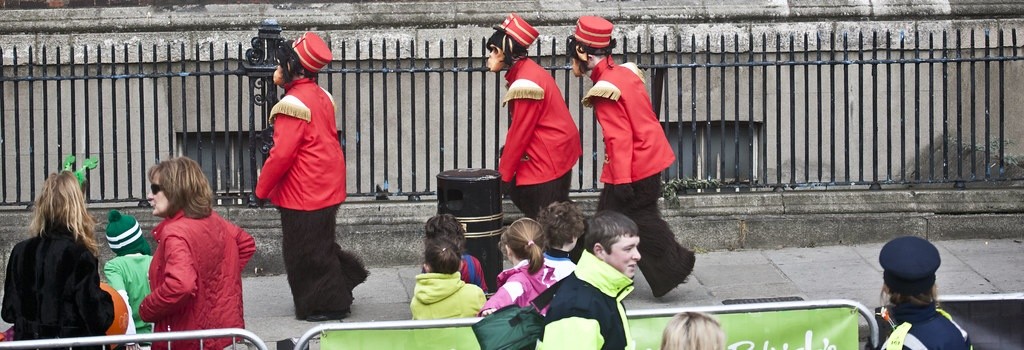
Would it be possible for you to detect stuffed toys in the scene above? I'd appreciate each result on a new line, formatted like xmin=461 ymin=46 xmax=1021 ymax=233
xmin=254 ymin=33 xmax=371 ymax=321
xmin=485 ymin=15 xmax=582 ymax=218
xmin=565 ymin=17 xmax=696 ymax=297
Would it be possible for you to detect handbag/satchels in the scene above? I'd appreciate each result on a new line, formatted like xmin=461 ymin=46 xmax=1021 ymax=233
xmin=472 ymin=278 xmax=566 ymax=350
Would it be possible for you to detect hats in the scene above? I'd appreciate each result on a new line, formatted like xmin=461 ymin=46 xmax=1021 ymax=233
xmin=494 ymin=14 xmax=539 ymax=49
xmin=105 ymin=210 xmax=151 ymax=255
xmin=879 ymin=237 xmax=941 ymax=292
xmin=574 ymin=16 xmax=614 ymax=48
xmin=292 ymin=32 xmax=332 ymax=73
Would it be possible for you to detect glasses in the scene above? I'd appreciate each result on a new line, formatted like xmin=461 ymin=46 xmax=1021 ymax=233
xmin=151 ymin=183 xmax=163 ymax=195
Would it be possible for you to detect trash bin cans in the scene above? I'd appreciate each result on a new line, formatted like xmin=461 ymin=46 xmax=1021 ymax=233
xmin=436 ymin=169 xmax=503 ymax=299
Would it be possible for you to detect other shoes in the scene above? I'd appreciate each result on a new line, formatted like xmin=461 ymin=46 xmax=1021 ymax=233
xmin=308 ymin=310 xmax=348 ymax=321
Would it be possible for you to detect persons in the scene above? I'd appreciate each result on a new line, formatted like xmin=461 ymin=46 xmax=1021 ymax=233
xmin=103 ymin=209 xmax=154 ymax=350
xmin=140 ymin=157 xmax=255 ymax=350
xmin=535 ymin=211 xmax=641 ymax=350
xmin=411 ymin=201 xmax=586 ymax=320
xmin=660 ymin=312 xmax=727 ymax=350
xmin=1 ymin=170 xmax=115 ymax=350
xmin=863 ymin=236 xmax=973 ymax=350
